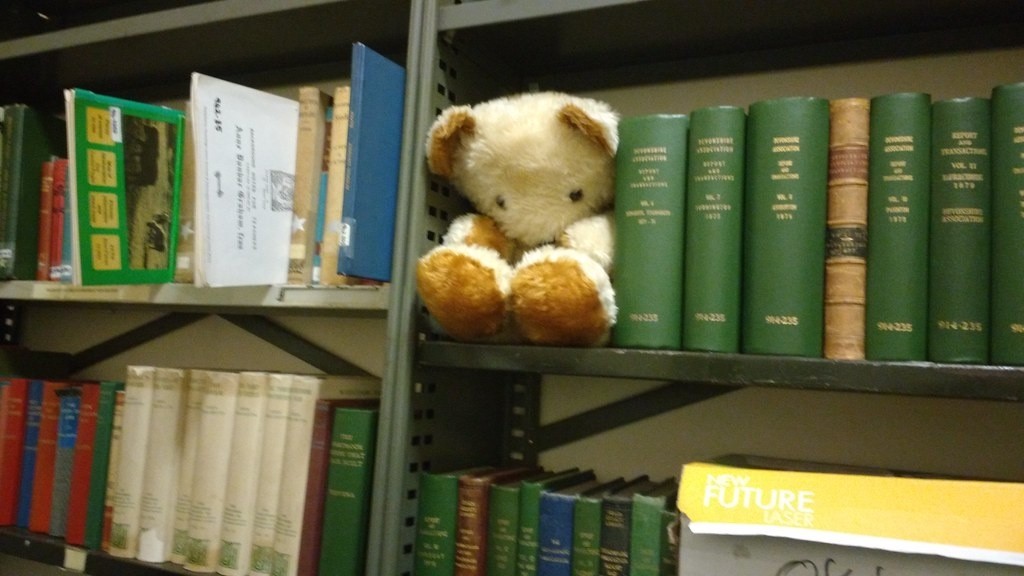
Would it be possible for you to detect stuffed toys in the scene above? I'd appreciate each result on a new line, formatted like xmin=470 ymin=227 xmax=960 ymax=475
xmin=415 ymin=92 xmax=622 ymax=347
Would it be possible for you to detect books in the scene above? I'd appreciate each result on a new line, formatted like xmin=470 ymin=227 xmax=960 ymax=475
xmin=615 ymin=81 xmax=1024 ymax=367
xmin=0 ymin=365 xmax=381 ymax=576
xmin=0 ymin=43 xmax=407 ymax=288
xmin=411 ymin=458 xmax=679 ymax=576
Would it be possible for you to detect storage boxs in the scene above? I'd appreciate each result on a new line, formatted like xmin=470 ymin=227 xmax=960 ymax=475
xmin=676 ymin=452 xmax=1024 ymax=576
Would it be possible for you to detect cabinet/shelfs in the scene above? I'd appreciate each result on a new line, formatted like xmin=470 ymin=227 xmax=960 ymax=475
xmin=0 ymin=0 xmax=1024 ymax=576
